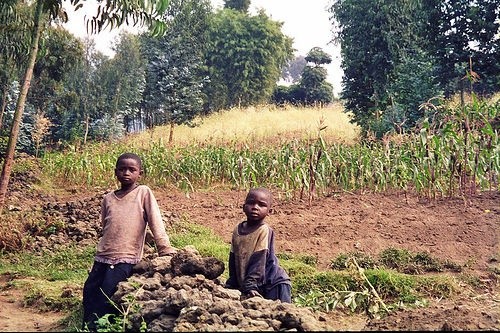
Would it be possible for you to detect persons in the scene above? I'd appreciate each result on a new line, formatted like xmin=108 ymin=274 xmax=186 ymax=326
xmin=82 ymin=153 xmax=176 ymax=331
xmin=220 ymin=186 xmax=292 ymax=304
